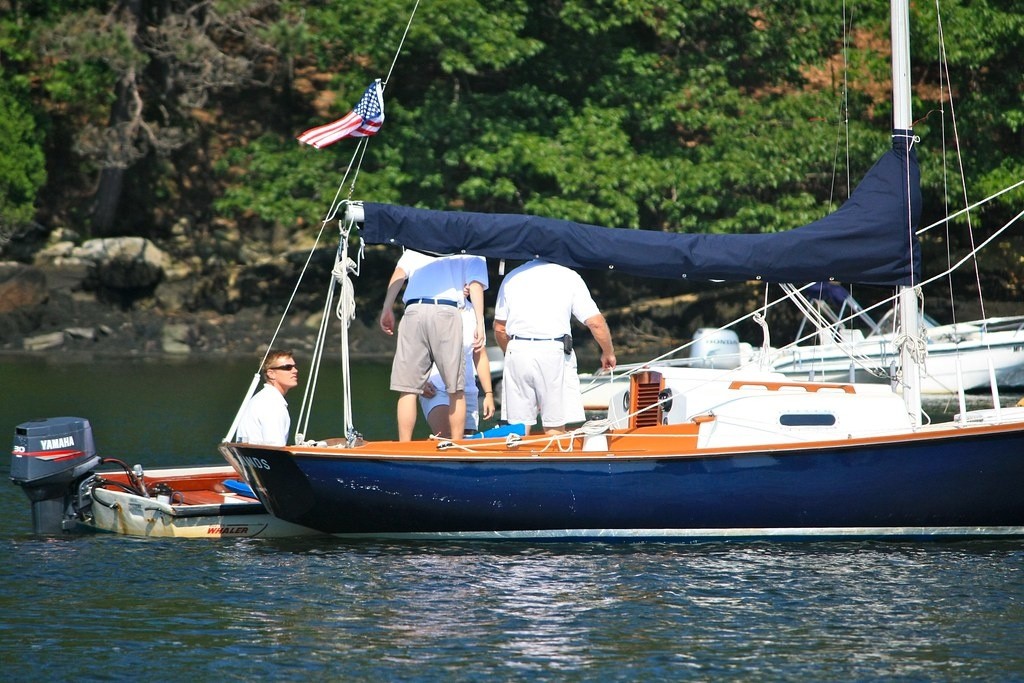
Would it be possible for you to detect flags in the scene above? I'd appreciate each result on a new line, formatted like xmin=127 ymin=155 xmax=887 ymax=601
xmin=296 ymin=80 xmax=382 ymax=149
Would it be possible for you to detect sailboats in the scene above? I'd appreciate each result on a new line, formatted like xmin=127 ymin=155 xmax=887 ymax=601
xmin=220 ymin=0 xmax=1024 ymax=533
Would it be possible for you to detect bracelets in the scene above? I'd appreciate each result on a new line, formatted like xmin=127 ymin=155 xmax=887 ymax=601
xmin=484 ymin=391 xmax=493 ymax=394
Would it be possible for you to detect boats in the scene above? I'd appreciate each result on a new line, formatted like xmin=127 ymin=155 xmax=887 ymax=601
xmin=475 ymin=287 xmax=1024 ymax=425
xmin=9 ymin=416 xmax=320 ymax=539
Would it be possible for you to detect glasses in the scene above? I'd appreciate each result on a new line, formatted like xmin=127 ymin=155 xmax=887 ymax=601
xmin=263 ymin=363 xmax=299 ymax=371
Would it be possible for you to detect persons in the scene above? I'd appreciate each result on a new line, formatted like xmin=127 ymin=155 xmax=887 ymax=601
xmin=418 ymin=297 xmax=495 ymax=439
xmin=493 ymin=258 xmax=617 ymax=437
xmin=237 ymin=349 xmax=298 ymax=447
xmin=380 ymin=248 xmax=489 ymax=442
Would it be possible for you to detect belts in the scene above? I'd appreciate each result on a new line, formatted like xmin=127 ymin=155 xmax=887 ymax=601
xmin=511 ymin=335 xmax=565 ymax=342
xmin=404 ymin=298 xmax=457 ymax=308
